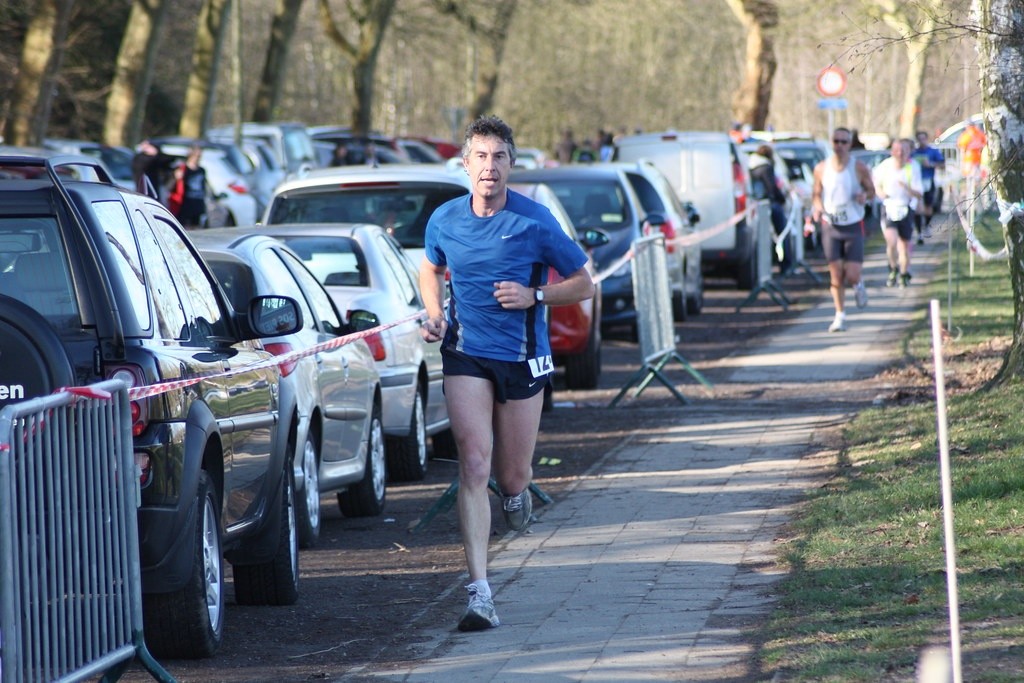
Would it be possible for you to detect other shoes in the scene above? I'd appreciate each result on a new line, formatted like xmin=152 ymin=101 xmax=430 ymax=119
xmin=924 ymin=228 xmax=932 ymax=237
xmin=917 ymin=239 xmax=923 ymax=245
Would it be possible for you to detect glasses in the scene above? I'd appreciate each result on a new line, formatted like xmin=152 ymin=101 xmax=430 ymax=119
xmin=833 ymin=138 xmax=849 ymax=145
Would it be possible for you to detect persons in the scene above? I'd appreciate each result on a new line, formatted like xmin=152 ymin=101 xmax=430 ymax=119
xmin=874 ymin=131 xmax=946 ymax=287
xmin=418 ymin=115 xmax=595 ymax=632
xmin=329 ymin=142 xmax=388 ymax=166
xmin=174 ymin=147 xmax=214 ymax=229
xmin=730 ymin=122 xmax=755 ymax=144
xmin=957 ymin=120 xmax=986 ymax=174
xmin=131 ymin=140 xmax=166 ymax=203
xmin=805 ymin=126 xmax=875 ymax=333
xmin=556 ymin=129 xmax=618 ymax=164
xmin=749 ymin=145 xmax=798 ymax=276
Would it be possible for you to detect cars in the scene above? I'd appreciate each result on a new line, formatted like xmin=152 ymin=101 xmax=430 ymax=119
xmin=0 ymin=111 xmax=998 ymax=415
xmin=255 ymin=156 xmax=469 ymax=287
xmin=182 ymin=229 xmax=388 ymax=550
xmin=228 ymin=221 xmax=452 ymax=486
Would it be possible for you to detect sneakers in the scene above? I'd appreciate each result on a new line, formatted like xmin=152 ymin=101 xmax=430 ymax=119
xmin=499 ymin=489 xmax=532 ymax=532
xmin=457 ymin=583 xmax=500 ymax=630
xmin=853 ymin=279 xmax=868 ymax=308
xmin=886 ymin=267 xmax=898 ymax=286
xmin=829 ymin=317 xmax=846 ymax=331
xmin=897 ymin=272 xmax=912 ymax=290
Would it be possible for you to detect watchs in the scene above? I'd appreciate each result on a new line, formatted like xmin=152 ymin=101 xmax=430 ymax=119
xmin=533 ymin=287 xmax=544 ymax=305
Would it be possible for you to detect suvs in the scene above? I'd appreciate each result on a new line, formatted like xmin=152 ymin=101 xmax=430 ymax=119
xmin=0 ymin=153 xmax=306 ymax=663
